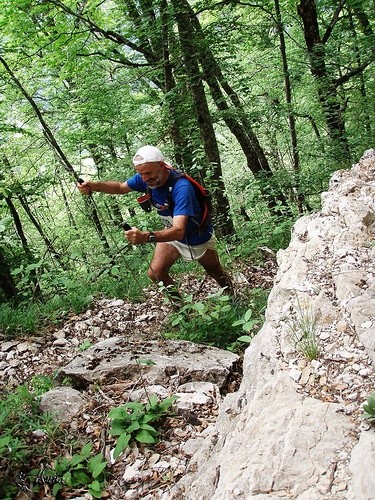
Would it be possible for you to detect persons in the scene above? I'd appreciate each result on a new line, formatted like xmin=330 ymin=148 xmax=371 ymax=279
xmin=75 ymin=145 xmax=236 ymax=311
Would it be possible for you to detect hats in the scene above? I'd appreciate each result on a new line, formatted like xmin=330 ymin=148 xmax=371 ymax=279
xmin=133 ymin=145 xmax=170 ymax=166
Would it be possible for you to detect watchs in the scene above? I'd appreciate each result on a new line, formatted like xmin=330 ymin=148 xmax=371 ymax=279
xmin=148 ymin=231 xmax=155 ymax=242
